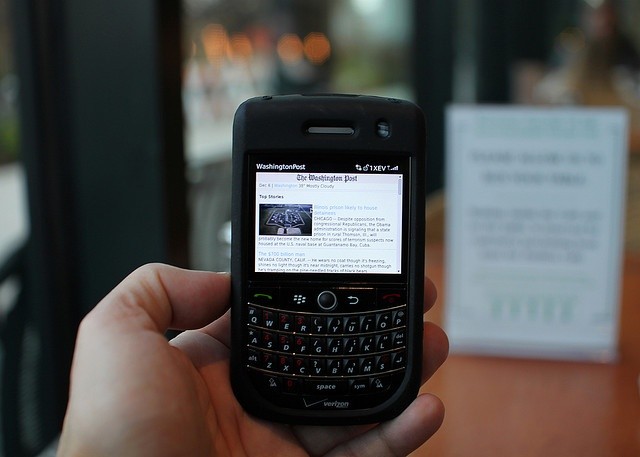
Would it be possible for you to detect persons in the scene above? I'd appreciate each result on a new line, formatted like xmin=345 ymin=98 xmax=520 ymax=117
xmin=56 ymin=262 xmax=450 ymax=457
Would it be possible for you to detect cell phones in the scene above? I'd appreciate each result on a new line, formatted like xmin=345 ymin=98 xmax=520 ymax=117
xmin=230 ymin=94 xmax=426 ymax=425
